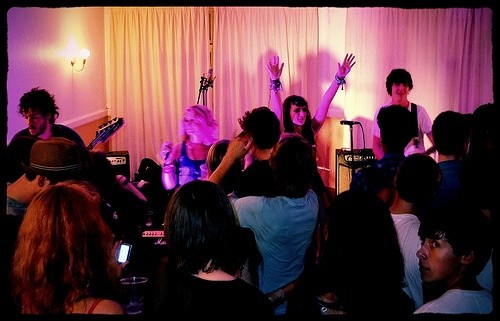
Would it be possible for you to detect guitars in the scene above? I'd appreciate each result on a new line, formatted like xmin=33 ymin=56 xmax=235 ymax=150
xmin=86 ymin=117 xmax=124 ymax=151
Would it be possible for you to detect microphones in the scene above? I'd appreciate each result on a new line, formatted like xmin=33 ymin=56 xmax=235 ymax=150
xmin=116 ymin=174 xmax=148 ymax=202
xmin=340 ymin=120 xmax=360 ymax=125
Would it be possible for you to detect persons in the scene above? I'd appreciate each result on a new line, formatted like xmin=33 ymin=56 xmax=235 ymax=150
xmin=12 ymin=180 xmax=128 ymax=315
xmin=267 ymin=52 xmax=359 ymax=204
xmin=206 ymin=139 xmax=246 ymax=181
xmin=227 ymin=132 xmax=324 ymax=316
xmin=7 ymin=137 xmax=94 ymax=247
xmin=140 ymin=178 xmax=277 ymax=316
xmin=160 ymin=103 xmax=225 ymax=193
xmin=6 ymin=87 xmax=86 ymax=184
xmin=315 ymin=101 xmax=495 ymax=314
xmin=209 ymin=104 xmax=285 ymax=198
xmin=370 ymin=68 xmax=439 ymax=164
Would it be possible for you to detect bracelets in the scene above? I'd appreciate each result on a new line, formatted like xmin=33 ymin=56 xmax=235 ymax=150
xmin=162 ymin=162 xmax=177 ymax=173
xmin=334 ymin=74 xmax=346 ymax=91
xmin=269 ymin=78 xmax=282 ymax=93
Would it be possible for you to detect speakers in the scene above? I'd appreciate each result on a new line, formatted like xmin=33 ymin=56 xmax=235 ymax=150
xmin=334 ymin=149 xmax=373 ymax=196
xmin=103 ymin=151 xmax=131 ymax=180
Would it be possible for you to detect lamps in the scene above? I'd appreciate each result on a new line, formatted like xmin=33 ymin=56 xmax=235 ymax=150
xmin=71 ymin=45 xmax=91 ymax=73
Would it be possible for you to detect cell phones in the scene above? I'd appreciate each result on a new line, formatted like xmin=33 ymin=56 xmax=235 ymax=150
xmin=117 ymin=243 xmax=133 ymax=265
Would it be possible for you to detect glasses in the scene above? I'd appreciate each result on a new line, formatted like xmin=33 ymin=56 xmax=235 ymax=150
xmin=293 ymin=107 xmax=308 ymax=112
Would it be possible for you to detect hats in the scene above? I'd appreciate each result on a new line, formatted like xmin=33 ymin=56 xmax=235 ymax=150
xmin=20 ymin=136 xmax=89 ymax=174
